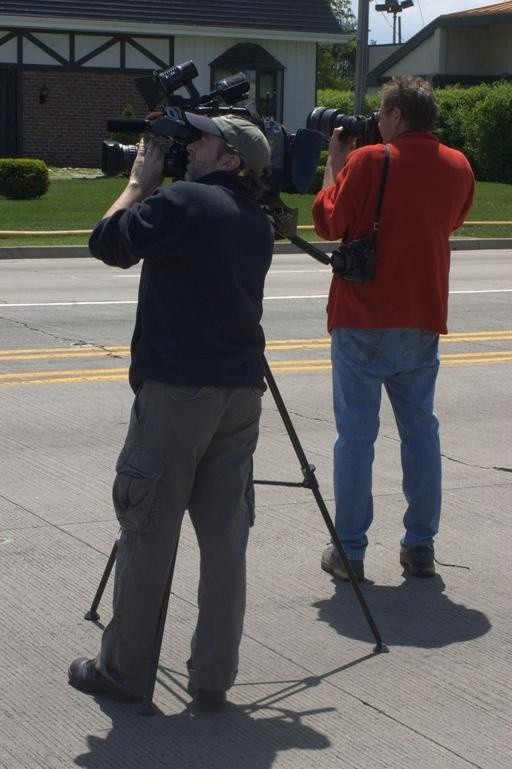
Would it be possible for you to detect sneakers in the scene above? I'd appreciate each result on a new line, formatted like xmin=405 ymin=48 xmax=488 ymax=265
xmin=68 ymin=657 xmax=138 ymax=701
xmin=321 ymin=546 xmax=364 ymax=581
xmin=187 ymin=679 xmax=226 ymax=710
xmin=399 ymin=540 xmax=436 ymax=578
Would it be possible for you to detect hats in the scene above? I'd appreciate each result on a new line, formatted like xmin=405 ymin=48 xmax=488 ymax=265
xmin=185 ymin=111 xmax=272 ymax=168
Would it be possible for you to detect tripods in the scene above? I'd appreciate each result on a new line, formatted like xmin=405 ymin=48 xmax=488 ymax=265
xmin=79 ymin=265 xmax=388 ymax=663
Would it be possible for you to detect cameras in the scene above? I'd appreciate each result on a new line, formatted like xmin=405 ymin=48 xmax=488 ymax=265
xmin=303 ymin=104 xmax=382 ymax=145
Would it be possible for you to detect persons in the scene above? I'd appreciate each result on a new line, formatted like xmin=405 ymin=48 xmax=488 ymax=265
xmin=311 ymin=74 xmax=476 ymax=582
xmin=68 ymin=111 xmax=277 ymax=706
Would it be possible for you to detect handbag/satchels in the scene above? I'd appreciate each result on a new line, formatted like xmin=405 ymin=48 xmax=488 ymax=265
xmin=331 ymin=238 xmax=378 ymax=281
